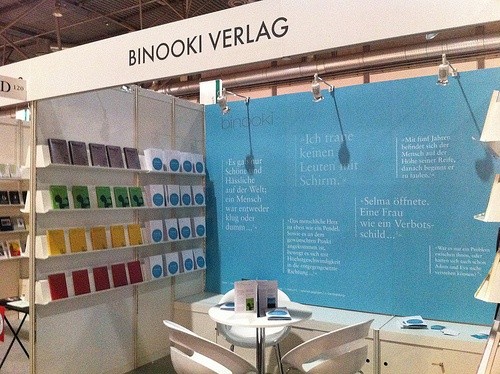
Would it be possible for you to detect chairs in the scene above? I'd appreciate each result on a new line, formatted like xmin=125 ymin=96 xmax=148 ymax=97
xmin=162 ymin=288 xmax=375 ymax=374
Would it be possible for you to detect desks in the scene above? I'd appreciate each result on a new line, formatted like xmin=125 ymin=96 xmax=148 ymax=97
xmin=0 ymin=278 xmax=29 ymax=370
xmin=208 ymin=301 xmax=312 ymax=374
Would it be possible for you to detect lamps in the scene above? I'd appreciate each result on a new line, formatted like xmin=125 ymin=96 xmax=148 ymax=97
xmin=217 ymin=88 xmax=249 ymax=114
xmin=311 ymin=72 xmax=334 ymax=102
xmin=435 ymin=53 xmax=457 ymax=86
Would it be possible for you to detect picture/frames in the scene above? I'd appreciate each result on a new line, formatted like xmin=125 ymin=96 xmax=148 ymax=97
xmin=6 ymin=240 xmax=22 ymax=257
xmin=0 ymin=216 xmax=14 ymax=231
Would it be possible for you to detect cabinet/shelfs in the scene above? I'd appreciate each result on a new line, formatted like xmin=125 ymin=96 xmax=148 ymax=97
xmin=172 ymin=290 xmax=493 ymax=374
xmin=0 ymin=144 xmax=206 ymax=306
